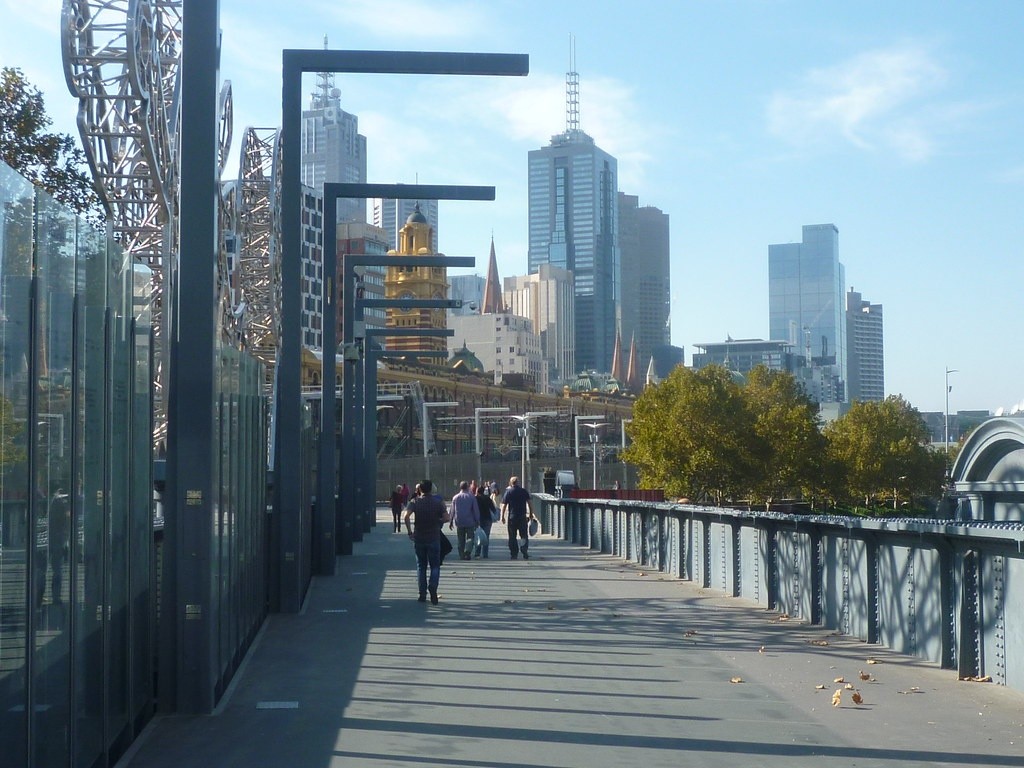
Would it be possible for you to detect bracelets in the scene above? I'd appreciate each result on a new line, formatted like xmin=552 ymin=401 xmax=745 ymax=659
xmin=408 ymin=532 xmax=412 ymax=535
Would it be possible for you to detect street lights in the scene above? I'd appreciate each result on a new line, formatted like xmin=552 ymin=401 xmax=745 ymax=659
xmin=503 ymin=416 xmax=540 ymax=489
xmin=580 ymin=423 xmax=610 ymax=490
xmin=946 ymin=369 xmax=958 ymax=479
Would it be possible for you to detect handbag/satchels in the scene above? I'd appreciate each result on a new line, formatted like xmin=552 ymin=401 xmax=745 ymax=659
xmin=389 ymin=493 xmax=394 ymax=508
xmin=529 ymin=518 xmax=538 ymax=536
xmin=474 ymin=525 xmax=488 ymax=547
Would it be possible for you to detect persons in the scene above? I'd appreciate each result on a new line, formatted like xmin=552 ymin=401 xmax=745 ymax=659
xmin=401 ymin=479 xmax=448 ymax=605
xmin=449 ymin=480 xmax=500 ymax=560
xmin=501 ymin=477 xmax=534 ymax=559
xmin=390 ymin=485 xmax=403 ymax=533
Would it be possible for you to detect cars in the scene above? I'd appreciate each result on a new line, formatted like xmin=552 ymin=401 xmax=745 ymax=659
xmin=152 ymin=459 xmax=166 ymax=532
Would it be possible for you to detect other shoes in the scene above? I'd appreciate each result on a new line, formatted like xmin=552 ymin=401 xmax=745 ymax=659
xmin=521 ymin=548 xmax=528 ymax=559
xmin=510 ymin=555 xmax=518 ymax=559
xmin=418 ymin=594 xmax=427 ymax=602
xmin=429 ymin=588 xmax=438 ymax=605
xmin=465 ymin=554 xmax=471 ymax=560
xmin=475 ymin=554 xmax=480 ymax=560
